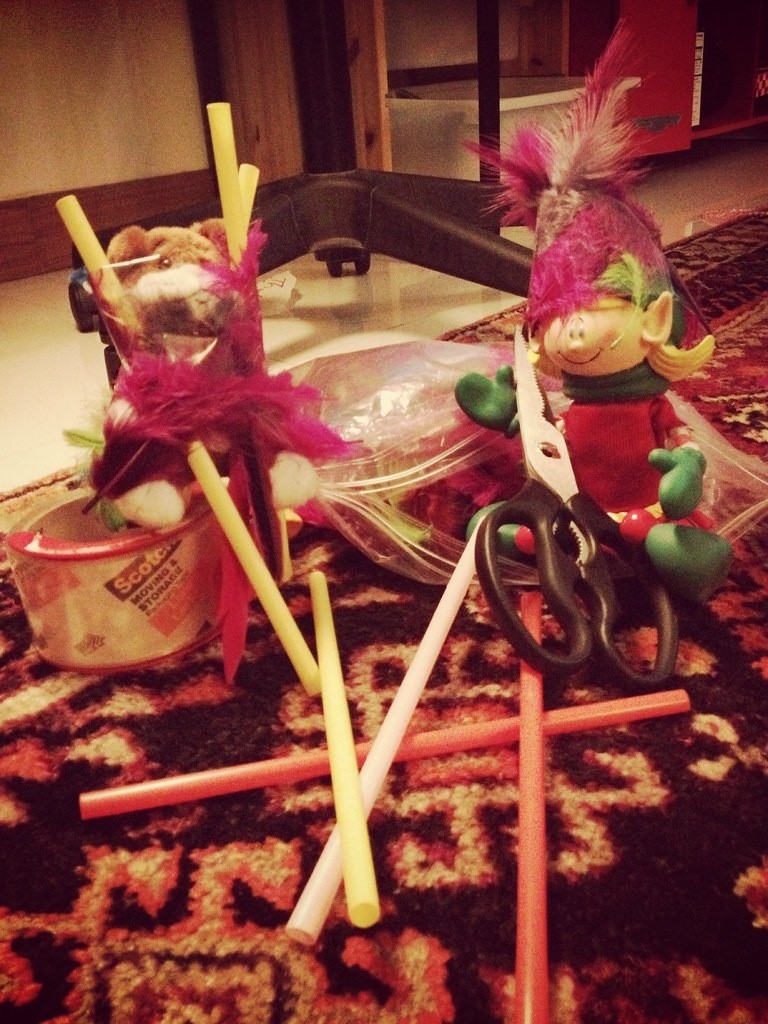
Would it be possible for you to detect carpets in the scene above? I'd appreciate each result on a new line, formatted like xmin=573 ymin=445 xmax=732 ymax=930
xmin=0 ymin=203 xmax=768 ymax=1024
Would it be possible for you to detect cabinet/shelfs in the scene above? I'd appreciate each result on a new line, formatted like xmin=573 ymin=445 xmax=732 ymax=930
xmin=212 ymin=0 xmax=768 ymax=186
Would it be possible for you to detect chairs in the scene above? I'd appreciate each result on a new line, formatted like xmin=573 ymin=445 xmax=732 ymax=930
xmin=70 ymin=0 xmax=535 ymax=383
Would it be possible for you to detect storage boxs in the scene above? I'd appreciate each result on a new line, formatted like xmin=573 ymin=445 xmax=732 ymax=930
xmin=389 ymin=74 xmax=643 ymax=180
